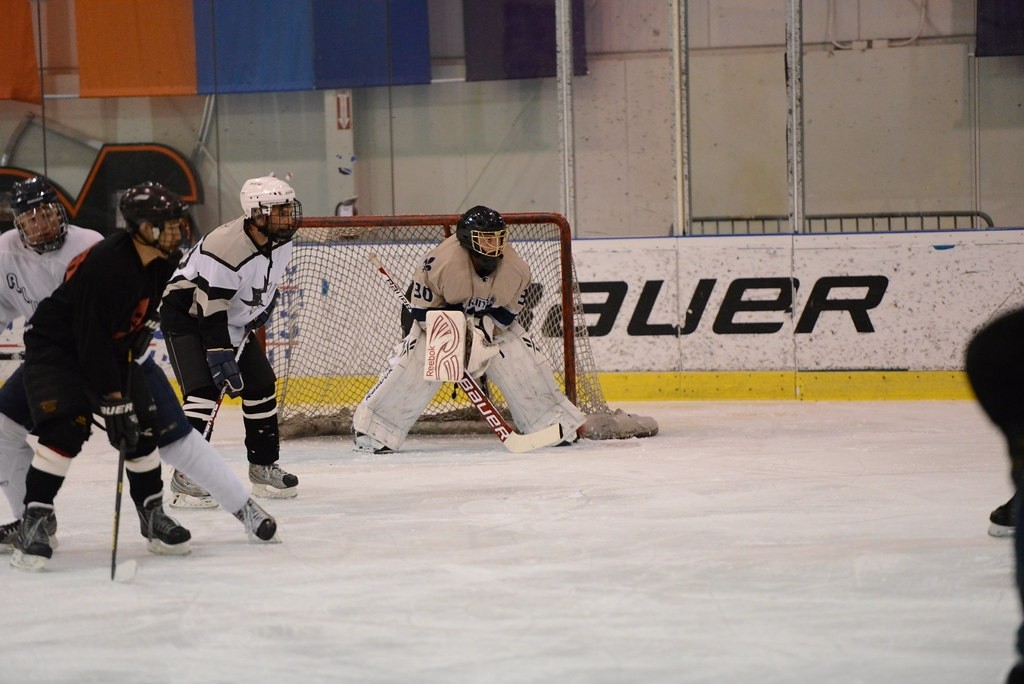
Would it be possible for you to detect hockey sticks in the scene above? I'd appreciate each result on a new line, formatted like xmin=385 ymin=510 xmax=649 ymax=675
xmin=110 ymin=341 xmax=136 ymax=584
xmin=0 ymin=350 xmax=26 ymax=361
xmin=202 ymin=324 xmax=254 ymax=442
xmin=366 ymin=252 xmax=565 ymax=453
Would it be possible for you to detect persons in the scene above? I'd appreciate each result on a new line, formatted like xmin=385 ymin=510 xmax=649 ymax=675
xmin=964 ymin=307 xmax=1024 ymax=683
xmin=351 ymin=205 xmax=588 ymax=454
xmin=12 ymin=180 xmax=200 ymax=559
xmin=159 ymin=176 xmax=303 ymax=498
xmin=0 ymin=176 xmax=278 ymax=546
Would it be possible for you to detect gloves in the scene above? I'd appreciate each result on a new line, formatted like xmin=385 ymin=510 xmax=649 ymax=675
xmin=244 ymin=288 xmax=281 ymax=330
xmin=126 ymin=311 xmax=160 ymax=359
xmin=100 ymin=397 xmax=139 ymax=449
xmin=206 ymin=348 xmax=245 ymax=399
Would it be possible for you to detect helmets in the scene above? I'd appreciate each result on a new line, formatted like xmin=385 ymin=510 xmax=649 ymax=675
xmin=119 ymin=181 xmax=194 ymax=260
xmin=9 ymin=176 xmax=68 ymax=254
xmin=455 ymin=205 xmax=507 ymax=257
xmin=240 ymin=176 xmax=303 ymax=242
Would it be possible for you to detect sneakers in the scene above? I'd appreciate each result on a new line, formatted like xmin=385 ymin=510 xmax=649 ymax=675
xmin=10 ymin=502 xmax=56 ymax=570
xmin=168 ymin=467 xmax=219 ymax=509
xmin=248 ymin=463 xmax=298 ymax=499
xmin=130 ymin=481 xmax=192 ymax=556
xmin=231 ymin=497 xmax=283 ymax=543
xmin=0 ymin=512 xmax=59 ymax=555
xmin=987 ymin=492 xmax=1018 ymax=536
